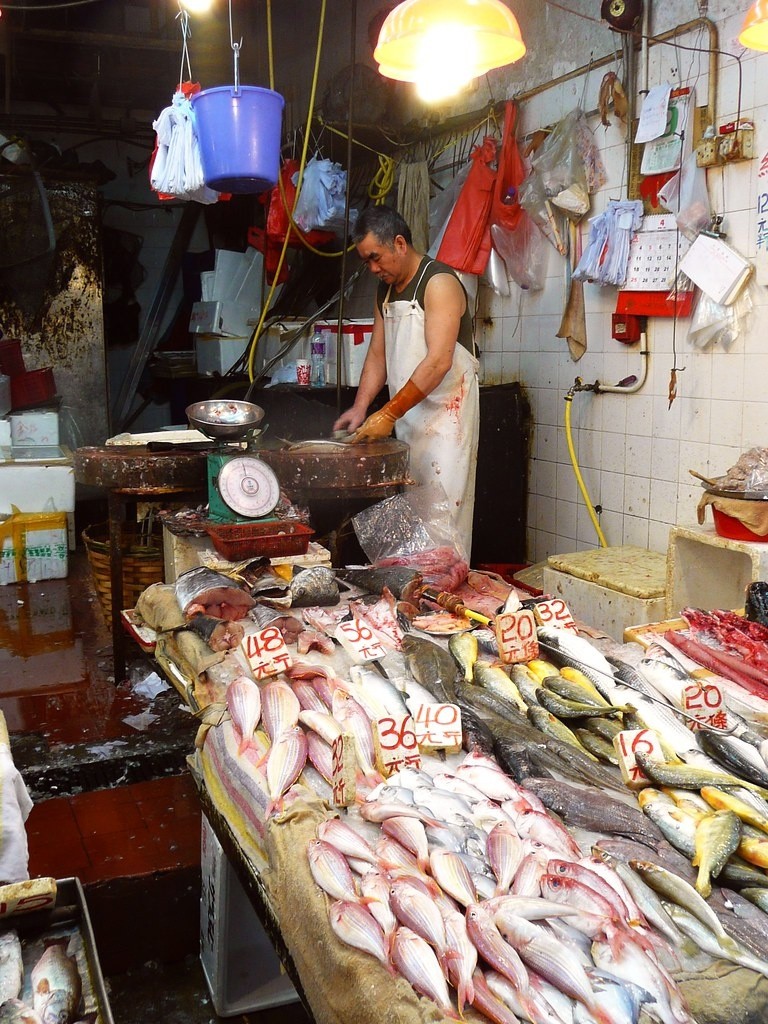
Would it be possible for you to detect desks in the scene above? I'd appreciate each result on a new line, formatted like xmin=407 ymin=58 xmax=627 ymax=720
xmin=182 ymin=611 xmax=768 ymax=1024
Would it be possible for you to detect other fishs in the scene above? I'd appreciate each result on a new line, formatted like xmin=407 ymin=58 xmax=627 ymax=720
xmin=175 ymin=543 xmax=768 ymax=1024
xmin=0 ymin=924 xmax=80 ymax=1024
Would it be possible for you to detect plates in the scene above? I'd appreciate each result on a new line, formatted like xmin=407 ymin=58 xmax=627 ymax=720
xmin=702 ymin=476 xmax=768 ymax=500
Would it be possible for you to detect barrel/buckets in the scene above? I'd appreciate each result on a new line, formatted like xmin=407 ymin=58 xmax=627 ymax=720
xmin=192 ymin=87 xmax=286 ymax=194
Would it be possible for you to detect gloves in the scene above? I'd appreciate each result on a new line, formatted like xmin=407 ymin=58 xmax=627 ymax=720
xmin=353 ymin=380 xmax=426 ymax=444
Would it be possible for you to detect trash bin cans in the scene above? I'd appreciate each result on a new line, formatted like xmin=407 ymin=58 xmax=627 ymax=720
xmin=78 ymin=519 xmax=164 ymax=632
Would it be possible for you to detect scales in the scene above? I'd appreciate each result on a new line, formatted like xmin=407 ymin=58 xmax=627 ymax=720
xmin=187 ymin=399 xmax=280 ymax=531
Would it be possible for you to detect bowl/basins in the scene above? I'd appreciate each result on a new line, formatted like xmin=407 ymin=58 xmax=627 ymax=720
xmin=711 ymin=503 xmax=768 ymax=542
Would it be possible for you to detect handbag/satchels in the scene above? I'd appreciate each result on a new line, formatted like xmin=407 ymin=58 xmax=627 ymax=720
xmin=266 ymin=158 xmax=357 ymax=246
xmin=148 ymin=81 xmax=233 ymax=201
xmin=426 ymin=101 xmax=644 ymax=317
xmin=657 ymin=148 xmax=712 ymax=241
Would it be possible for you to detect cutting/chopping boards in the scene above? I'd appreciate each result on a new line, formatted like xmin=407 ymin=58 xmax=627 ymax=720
xmin=74 ymin=444 xmax=218 ymax=488
xmin=263 ymin=429 xmax=411 ymax=501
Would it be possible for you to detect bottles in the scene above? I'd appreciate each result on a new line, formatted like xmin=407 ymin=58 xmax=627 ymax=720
xmin=310 ymin=328 xmax=326 ymax=386
xmin=504 ymin=188 xmax=515 ymax=204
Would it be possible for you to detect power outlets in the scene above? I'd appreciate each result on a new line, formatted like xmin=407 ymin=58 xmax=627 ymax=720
xmin=696 ymin=126 xmax=757 ymax=169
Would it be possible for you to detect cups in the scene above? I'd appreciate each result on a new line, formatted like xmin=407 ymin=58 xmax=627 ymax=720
xmin=296 ymin=359 xmax=311 ymax=386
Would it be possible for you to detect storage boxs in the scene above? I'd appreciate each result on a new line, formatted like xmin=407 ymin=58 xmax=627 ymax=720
xmin=542 ymin=521 xmax=768 ymax=645
xmin=0 ymin=408 xmax=76 ymax=587
xmin=188 ymin=301 xmax=376 ymax=387
xmin=163 ymin=522 xmax=334 ymax=582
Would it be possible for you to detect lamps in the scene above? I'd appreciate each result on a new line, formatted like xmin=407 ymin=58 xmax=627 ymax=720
xmin=738 ymin=0 xmax=768 ymax=54
xmin=374 ymin=0 xmax=528 ymax=85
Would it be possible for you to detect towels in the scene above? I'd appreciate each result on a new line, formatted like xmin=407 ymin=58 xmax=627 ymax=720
xmin=0 ymin=743 xmax=33 ymax=887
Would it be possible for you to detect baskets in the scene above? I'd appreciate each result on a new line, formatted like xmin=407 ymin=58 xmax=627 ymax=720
xmin=83 ymin=523 xmax=164 ymax=626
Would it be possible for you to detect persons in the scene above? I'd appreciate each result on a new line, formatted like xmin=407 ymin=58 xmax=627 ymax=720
xmin=333 ymin=204 xmax=481 ymax=573
